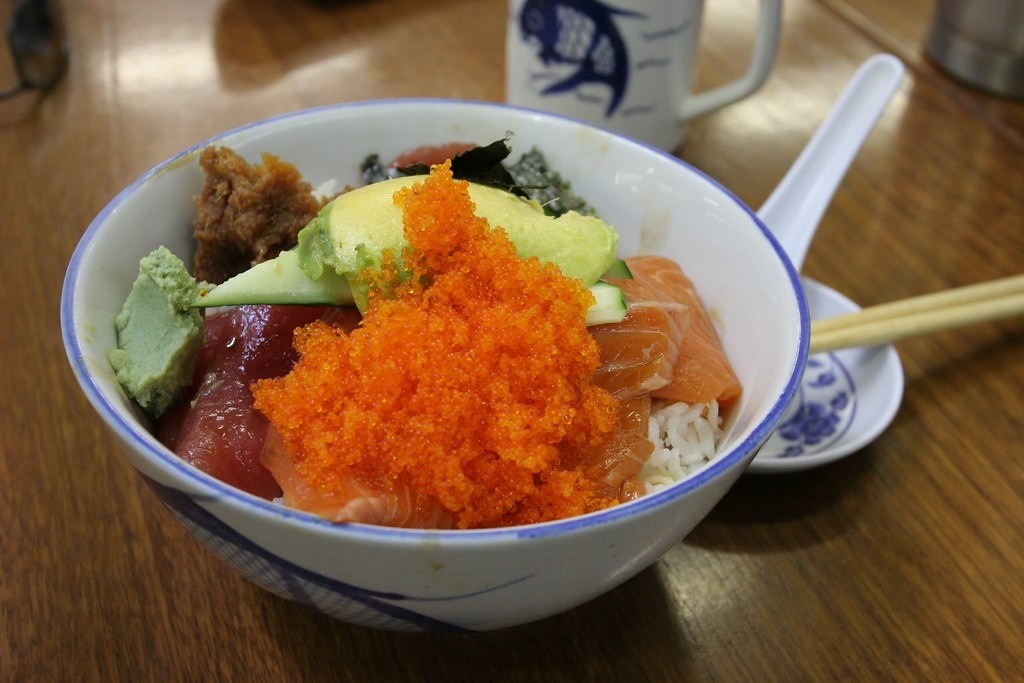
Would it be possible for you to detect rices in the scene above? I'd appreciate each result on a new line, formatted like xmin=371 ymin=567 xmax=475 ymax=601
xmin=637 ymin=397 xmax=724 ymax=494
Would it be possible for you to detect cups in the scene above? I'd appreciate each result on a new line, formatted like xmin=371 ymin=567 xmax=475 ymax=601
xmin=506 ymin=1 xmax=781 ymax=154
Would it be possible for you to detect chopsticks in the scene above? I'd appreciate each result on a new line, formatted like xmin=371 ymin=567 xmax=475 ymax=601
xmin=805 ymin=274 xmax=1022 ymax=354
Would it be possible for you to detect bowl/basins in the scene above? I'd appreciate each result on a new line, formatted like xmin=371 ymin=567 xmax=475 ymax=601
xmin=62 ymin=99 xmax=809 ymax=634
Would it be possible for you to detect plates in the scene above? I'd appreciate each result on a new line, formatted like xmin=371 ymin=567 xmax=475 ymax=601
xmin=742 ymin=279 xmax=903 ymax=471
